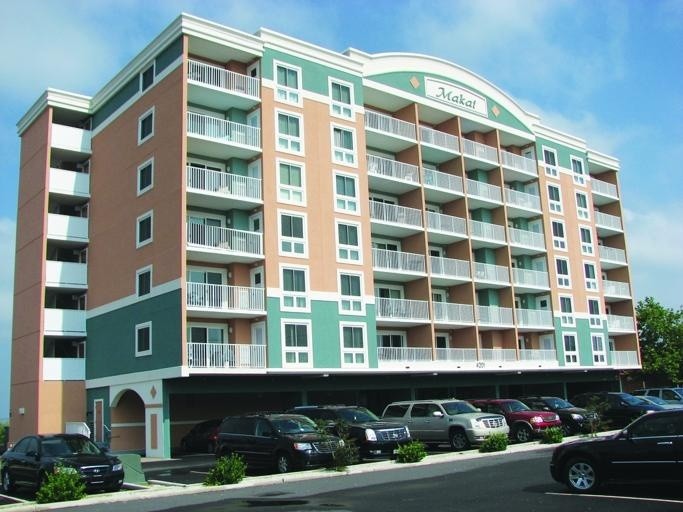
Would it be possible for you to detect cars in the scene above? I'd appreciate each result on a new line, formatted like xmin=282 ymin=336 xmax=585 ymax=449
xmin=548 ymin=406 xmax=683 ymax=494
xmin=0 ymin=431 xmax=125 ymax=499
xmin=177 ymin=417 xmax=222 ymax=454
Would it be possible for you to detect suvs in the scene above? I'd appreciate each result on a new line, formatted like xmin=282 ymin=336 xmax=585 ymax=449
xmin=282 ymin=403 xmax=416 ymax=464
xmin=469 ymin=383 xmax=683 ymax=444
xmin=379 ymin=398 xmax=512 ymax=451
xmin=212 ymin=409 xmax=352 ymax=478
